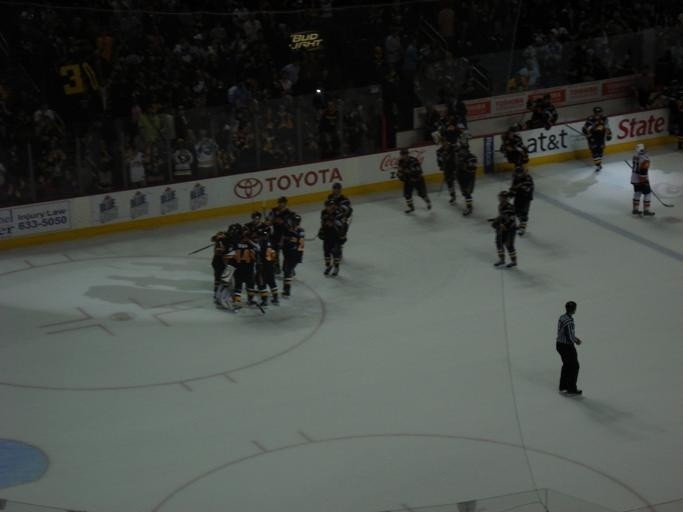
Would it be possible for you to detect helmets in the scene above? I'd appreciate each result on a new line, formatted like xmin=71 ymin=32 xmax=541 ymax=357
xmin=333 ymin=183 xmax=341 ymax=189
xmin=593 ymin=107 xmax=603 ymax=113
xmin=399 ymin=149 xmax=408 ymax=154
xmin=635 ymin=143 xmax=645 ymax=154
xmin=278 ymin=197 xmax=287 ymax=202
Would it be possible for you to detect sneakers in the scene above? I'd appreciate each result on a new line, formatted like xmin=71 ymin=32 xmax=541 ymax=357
xmin=632 ymin=209 xmax=641 ymax=213
xmin=247 ymin=291 xmax=290 ymax=306
xmin=558 ymin=387 xmax=582 ymax=394
xmin=494 ymin=261 xmax=516 ymax=268
xmin=464 ymin=206 xmax=471 ymax=215
xmin=645 ymin=210 xmax=655 ymax=216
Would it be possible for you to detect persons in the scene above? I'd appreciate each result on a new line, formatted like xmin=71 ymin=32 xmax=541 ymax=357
xmin=456 ymin=142 xmax=477 ymax=216
xmin=507 ymin=165 xmax=534 ymax=235
xmin=631 ymin=144 xmax=656 ymax=216
xmin=397 ymin=149 xmax=432 ymax=213
xmin=1 ymin=2 xmax=681 ymax=208
xmin=489 ymin=191 xmax=517 ymax=268
xmin=319 ymin=183 xmax=353 ymax=276
xmin=556 ymin=302 xmax=583 ymax=395
xmin=211 ymin=196 xmax=304 ymax=308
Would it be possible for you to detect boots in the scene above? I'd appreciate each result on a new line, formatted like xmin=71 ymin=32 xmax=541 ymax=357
xmin=406 ymin=204 xmax=414 ymax=213
xmin=596 ymin=164 xmax=602 ymax=171
xmin=450 ymin=193 xmax=456 ymax=203
xmin=324 ymin=264 xmax=338 ymax=276
xmin=426 ymin=199 xmax=431 ymax=209
